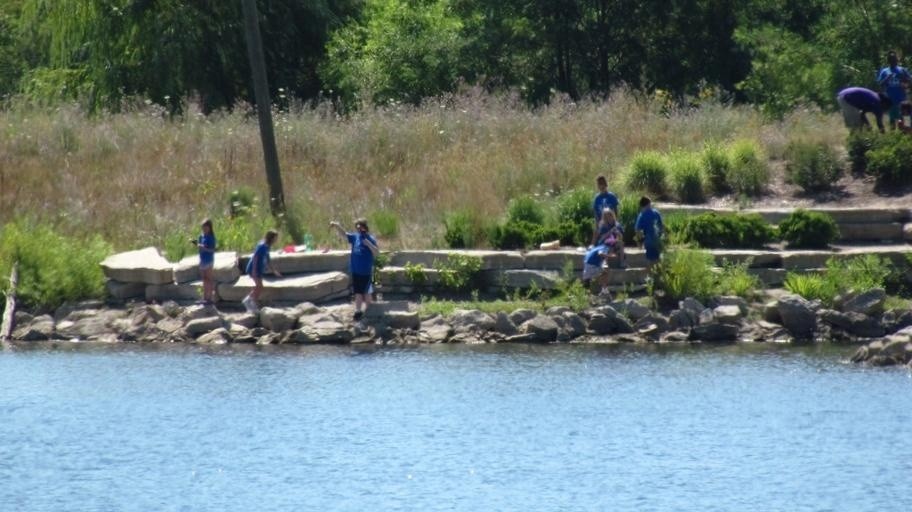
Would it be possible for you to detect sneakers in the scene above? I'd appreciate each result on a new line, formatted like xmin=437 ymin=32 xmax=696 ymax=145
xmin=242 ymin=297 xmax=260 ymax=314
xmin=194 ymin=297 xmax=214 ymax=304
xmin=351 ymin=310 xmax=363 ymax=321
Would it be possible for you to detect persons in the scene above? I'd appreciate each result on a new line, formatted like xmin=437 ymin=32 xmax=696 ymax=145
xmin=328 ymin=216 xmax=379 ymax=321
xmin=241 ymin=229 xmax=284 ymax=312
xmin=582 ymin=237 xmax=617 ymax=295
xmin=590 ymin=174 xmax=618 ymax=249
xmin=837 ymin=86 xmax=891 ymax=140
xmin=875 ymin=51 xmax=912 ymax=131
xmin=634 ymin=195 xmax=665 ymax=278
xmin=598 ymin=207 xmax=629 ymax=269
xmin=189 ymin=217 xmax=217 ymax=304
